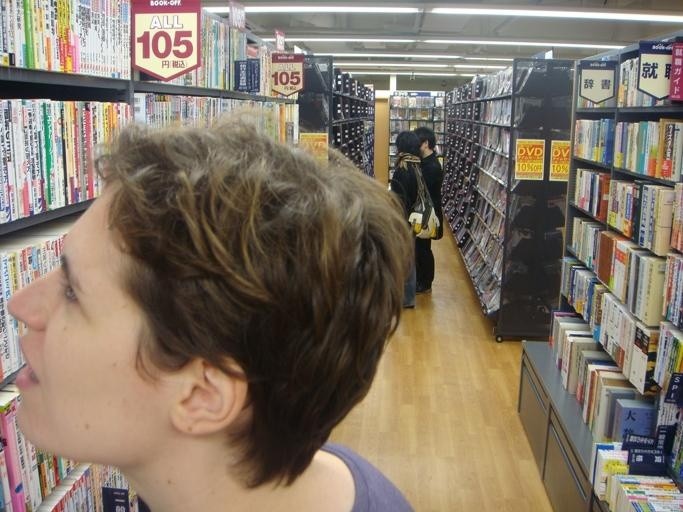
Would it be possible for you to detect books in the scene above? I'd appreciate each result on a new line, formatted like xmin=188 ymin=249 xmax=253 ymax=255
xmin=387 ymin=43 xmax=681 ymax=512
xmin=0 ymin=2 xmax=374 ymax=512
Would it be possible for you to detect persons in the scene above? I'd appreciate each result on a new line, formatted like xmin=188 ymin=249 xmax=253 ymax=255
xmin=388 ymin=126 xmax=446 ymax=298
xmin=7 ymin=116 xmax=418 ymax=512
